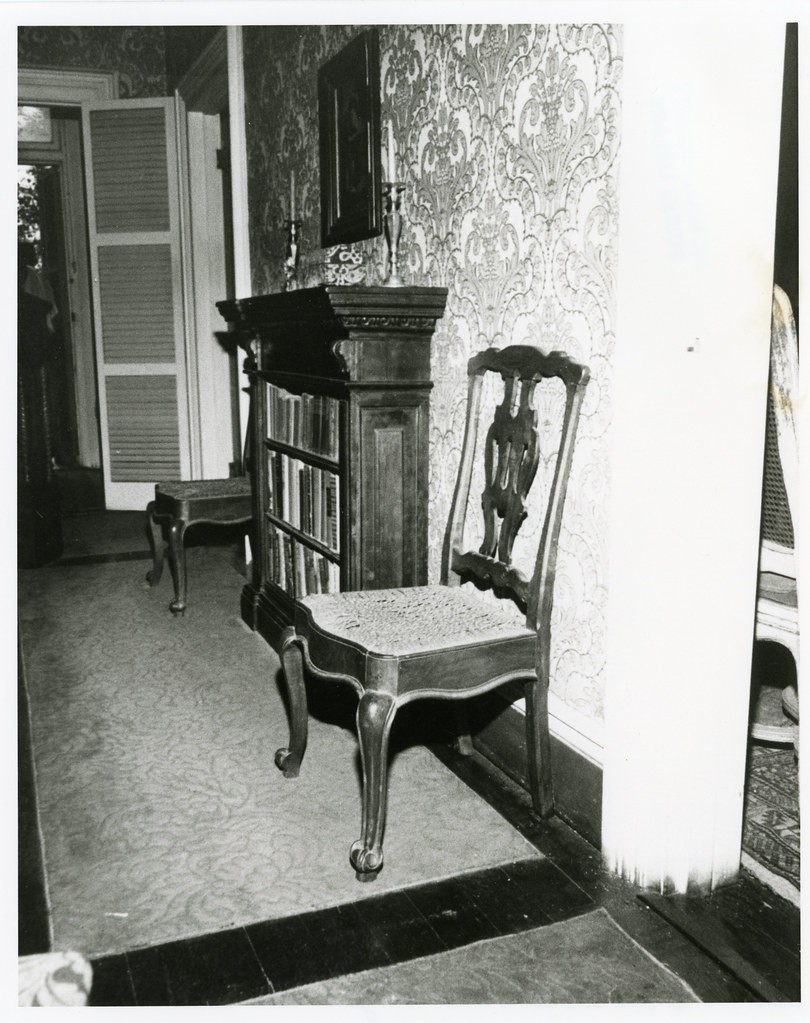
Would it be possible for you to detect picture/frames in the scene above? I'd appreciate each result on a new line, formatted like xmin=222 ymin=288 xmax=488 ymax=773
xmin=317 ymin=27 xmax=381 ymax=247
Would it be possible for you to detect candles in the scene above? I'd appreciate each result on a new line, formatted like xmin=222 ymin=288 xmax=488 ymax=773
xmin=290 ymin=170 xmax=295 ymax=220
xmin=388 ymin=120 xmax=396 ymax=182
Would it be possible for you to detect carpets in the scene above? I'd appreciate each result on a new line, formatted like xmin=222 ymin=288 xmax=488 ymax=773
xmin=17 ymin=544 xmax=545 ymax=959
xmin=740 ymin=651 xmax=800 ymax=911
xmin=212 ymin=908 xmax=706 ymax=1003
xmin=62 ymin=509 xmax=169 ymax=557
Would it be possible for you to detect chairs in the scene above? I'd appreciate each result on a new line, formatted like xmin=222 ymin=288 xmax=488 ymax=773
xmin=750 ymin=282 xmax=800 ymax=743
xmin=276 ymin=346 xmax=590 ymax=882
xmin=145 ymin=388 xmax=253 ymax=613
